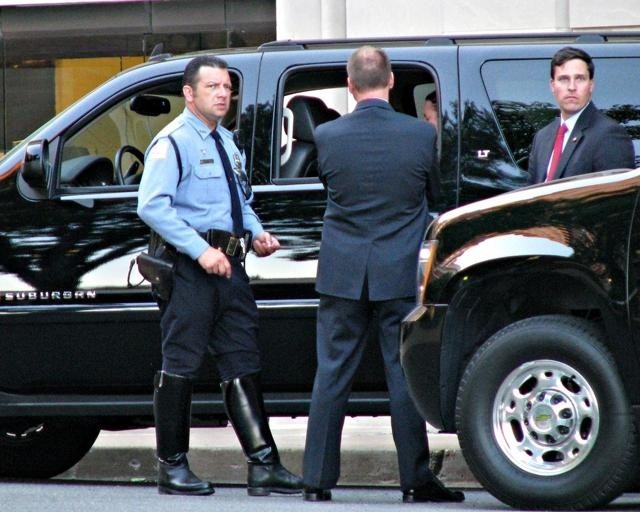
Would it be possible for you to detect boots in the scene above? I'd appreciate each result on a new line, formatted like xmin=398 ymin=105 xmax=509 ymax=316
xmin=153 ymin=370 xmax=215 ymax=495
xmin=220 ymin=375 xmax=304 ymax=496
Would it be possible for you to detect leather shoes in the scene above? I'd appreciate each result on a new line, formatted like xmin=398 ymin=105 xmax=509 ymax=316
xmin=304 ymin=489 xmax=331 ymax=501
xmin=403 ymin=476 xmax=464 ymax=502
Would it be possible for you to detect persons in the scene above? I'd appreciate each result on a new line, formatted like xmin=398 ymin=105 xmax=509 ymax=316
xmin=135 ymin=55 xmax=308 ymax=497
xmin=300 ymin=43 xmax=471 ymax=503
xmin=525 ymin=46 xmax=636 ymax=188
xmin=421 ymin=90 xmax=439 ymax=135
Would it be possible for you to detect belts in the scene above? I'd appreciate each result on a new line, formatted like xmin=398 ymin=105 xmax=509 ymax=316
xmin=202 ymin=232 xmax=252 ymax=258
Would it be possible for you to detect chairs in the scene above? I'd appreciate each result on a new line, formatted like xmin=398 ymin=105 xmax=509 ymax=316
xmin=280 ymin=94 xmax=341 ymax=180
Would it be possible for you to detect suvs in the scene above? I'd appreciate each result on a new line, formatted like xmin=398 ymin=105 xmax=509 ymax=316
xmin=394 ymin=163 xmax=640 ymax=511
xmin=0 ymin=32 xmax=640 ymax=498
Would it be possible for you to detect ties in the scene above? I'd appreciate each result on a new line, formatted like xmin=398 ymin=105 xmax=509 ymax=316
xmin=210 ymin=130 xmax=243 ymax=238
xmin=546 ymin=123 xmax=567 ymax=181
